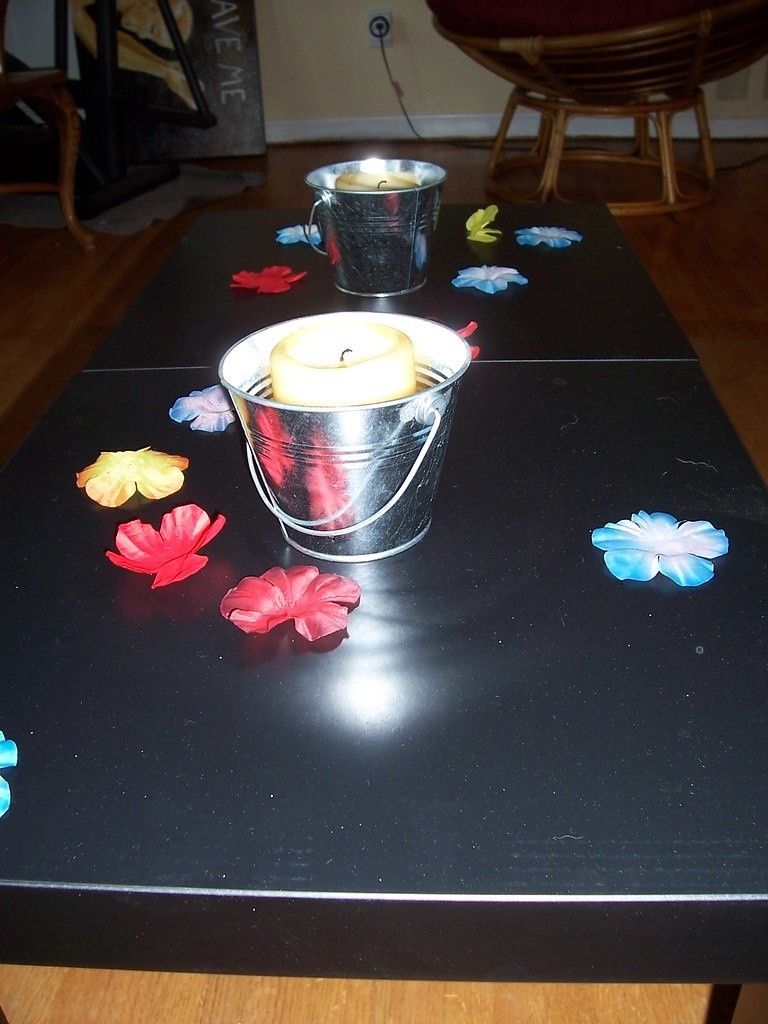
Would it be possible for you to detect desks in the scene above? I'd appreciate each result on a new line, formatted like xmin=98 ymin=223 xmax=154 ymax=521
xmin=0 ymin=202 xmax=768 ymax=1024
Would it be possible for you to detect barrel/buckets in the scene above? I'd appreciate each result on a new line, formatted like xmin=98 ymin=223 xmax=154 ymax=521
xmin=303 ymin=159 xmax=449 ymax=298
xmin=219 ymin=311 xmax=470 ymax=562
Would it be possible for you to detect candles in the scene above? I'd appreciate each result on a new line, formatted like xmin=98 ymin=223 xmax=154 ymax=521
xmin=268 ymin=324 xmax=417 ymax=405
xmin=336 ymin=172 xmax=417 ymax=190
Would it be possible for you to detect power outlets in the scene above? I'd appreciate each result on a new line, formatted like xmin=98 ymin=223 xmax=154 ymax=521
xmin=368 ymin=9 xmax=394 ymax=48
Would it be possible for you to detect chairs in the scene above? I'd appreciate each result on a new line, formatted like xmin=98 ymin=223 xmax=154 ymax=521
xmin=433 ymin=0 xmax=768 ymax=217
xmin=0 ymin=0 xmax=93 ymax=248
